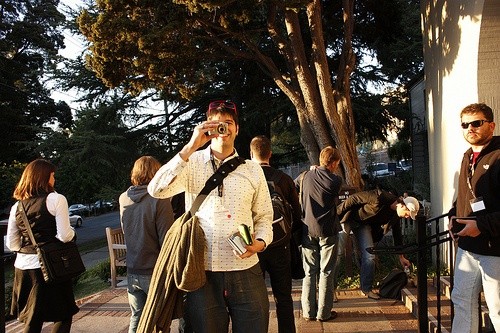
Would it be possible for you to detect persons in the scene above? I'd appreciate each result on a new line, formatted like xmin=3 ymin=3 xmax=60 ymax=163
xmin=4 ymin=159 xmax=80 ymax=333
xmin=336 ymin=187 xmax=419 ymax=300
xmin=147 ymin=99 xmax=275 ymax=333
xmin=449 ymin=102 xmax=500 ymax=333
xmin=292 ymin=145 xmax=342 ymax=321
xmin=245 ymin=136 xmax=302 ymax=333
xmin=117 ymin=155 xmax=177 ymax=333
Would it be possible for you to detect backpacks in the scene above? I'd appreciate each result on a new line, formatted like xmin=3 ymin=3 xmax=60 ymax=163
xmin=262 ymin=169 xmax=294 ymax=250
xmin=376 ymin=268 xmax=408 ymax=306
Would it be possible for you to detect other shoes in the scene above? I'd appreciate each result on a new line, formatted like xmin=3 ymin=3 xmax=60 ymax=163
xmin=366 ymin=290 xmax=380 ymax=300
xmin=318 ymin=311 xmax=337 ymax=322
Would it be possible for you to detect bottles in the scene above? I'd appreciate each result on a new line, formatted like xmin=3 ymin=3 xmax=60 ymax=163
xmin=404 ymin=266 xmax=410 ymax=277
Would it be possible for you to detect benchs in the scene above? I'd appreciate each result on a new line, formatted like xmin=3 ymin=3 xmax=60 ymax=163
xmin=106 ymin=227 xmax=128 ymax=289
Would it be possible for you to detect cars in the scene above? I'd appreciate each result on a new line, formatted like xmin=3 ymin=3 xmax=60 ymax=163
xmin=363 ymin=163 xmax=395 ymax=178
xmin=69 ymin=198 xmax=119 ymax=217
xmin=397 ymin=159 xmax=414 ymax=173
xmin=68 ymin=211 xmax=83 ymax=227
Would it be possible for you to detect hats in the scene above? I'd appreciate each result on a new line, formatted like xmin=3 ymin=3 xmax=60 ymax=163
xmin=403 ymin=196 xmax=419 ymax=220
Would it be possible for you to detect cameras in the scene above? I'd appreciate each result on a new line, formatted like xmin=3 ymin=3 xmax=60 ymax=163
xmin=209 ymin=122 xmax=228 ymax=136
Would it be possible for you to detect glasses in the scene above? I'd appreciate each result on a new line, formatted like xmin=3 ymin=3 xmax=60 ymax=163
xmin=461 ymin=118 xmax=492 ymax=129
xmin=208 ymin=100 xmax=237 ymax=115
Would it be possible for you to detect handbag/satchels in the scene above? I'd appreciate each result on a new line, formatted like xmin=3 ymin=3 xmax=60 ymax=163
xmin=36 ymin=240 xmax=86 ymax=283
xmin=172 ymin=215 xmax=207 ymax=292
